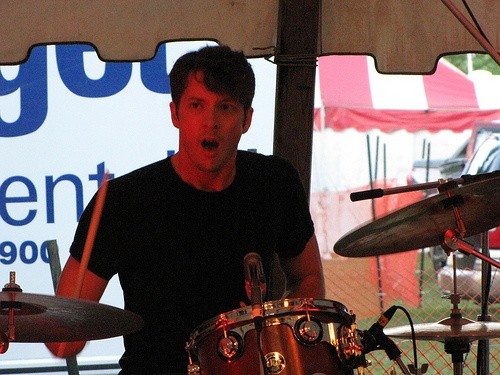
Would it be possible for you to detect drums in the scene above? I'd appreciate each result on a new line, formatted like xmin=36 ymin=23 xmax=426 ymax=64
xmin=183 ymin=297 xmax=372 ymax=375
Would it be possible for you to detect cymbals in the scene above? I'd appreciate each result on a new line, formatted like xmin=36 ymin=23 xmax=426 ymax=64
xmin=382 ymin=317 xmax=500 ymax=343
xmin=0 ymin=291 xmax=147 ymax=343
xmin=332 ymin=175 xmax=500 ymax=258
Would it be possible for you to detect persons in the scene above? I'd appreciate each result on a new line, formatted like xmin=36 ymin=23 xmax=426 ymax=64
xmin=43 ymin=46 xmax=326 ymax=375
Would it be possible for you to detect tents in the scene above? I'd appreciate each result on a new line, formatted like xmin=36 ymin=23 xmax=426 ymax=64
xmin=310 ymin=51 xmax=500 ymax=258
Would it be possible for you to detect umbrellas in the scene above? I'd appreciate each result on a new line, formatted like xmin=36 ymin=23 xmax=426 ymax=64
xmin=0 ymin=0 xmax=500 ymax=214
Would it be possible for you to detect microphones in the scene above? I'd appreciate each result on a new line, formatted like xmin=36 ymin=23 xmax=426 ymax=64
xmin=243 ymin=252 xmax=267 ymax=302
xmin=362 ymin=304 xmax=398 ymax=353
